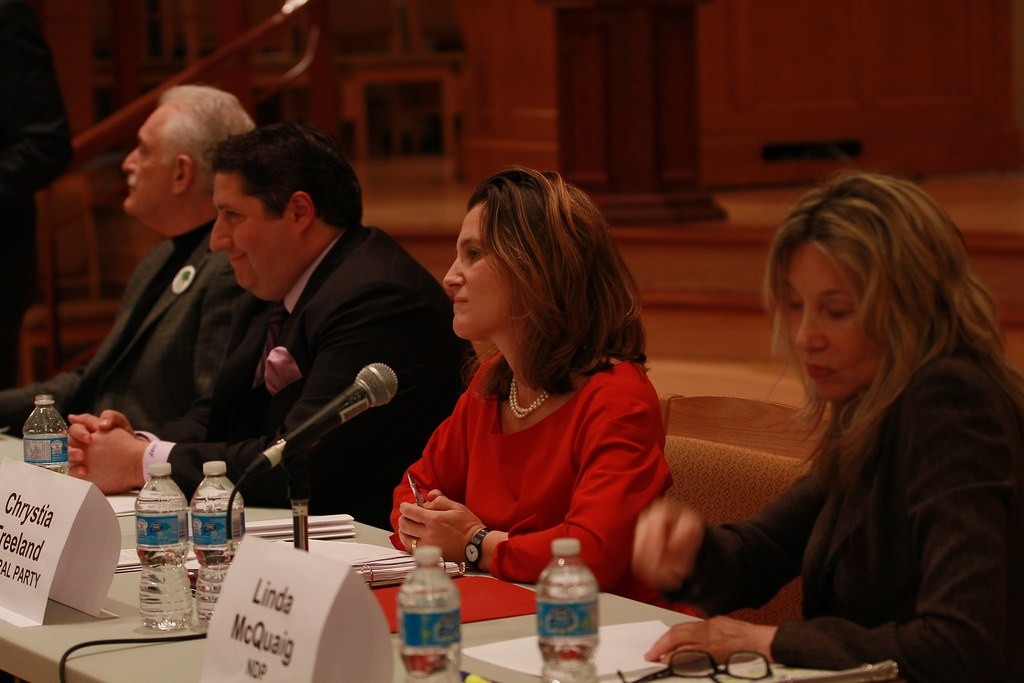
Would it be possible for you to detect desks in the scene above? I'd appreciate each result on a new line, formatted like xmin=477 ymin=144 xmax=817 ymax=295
xmin=0 ymin=433 xmax=899 ymax=683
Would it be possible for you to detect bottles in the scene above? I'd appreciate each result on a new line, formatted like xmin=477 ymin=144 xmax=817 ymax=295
xmin=190 ymin=460 xmax=246 ymax=630
xmin=23 ymin=394 xmax=70 ymax=476
xmin=394 ymin=544 xmax=464 ymax=683
xmin=534 ymin=537 xmax=601 ymax=683
xmin=134 ymin=462 xmax=193 ymax=633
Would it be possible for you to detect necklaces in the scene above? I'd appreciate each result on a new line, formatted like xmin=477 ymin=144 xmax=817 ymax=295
xmin=509 ymin=376 xmax=549 ymax=419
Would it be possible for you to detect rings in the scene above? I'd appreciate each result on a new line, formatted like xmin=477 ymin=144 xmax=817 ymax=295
xmin=411 ymin=538 xmax=418 ymax=551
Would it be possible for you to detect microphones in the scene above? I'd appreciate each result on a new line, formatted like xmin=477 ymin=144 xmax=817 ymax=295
xmin=245 ymin=363 xmax=398 ymax=480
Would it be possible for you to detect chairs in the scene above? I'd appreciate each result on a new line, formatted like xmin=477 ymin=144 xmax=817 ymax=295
xmin=342 ymin=0 xmax=492 ymax=192
xmin=657 ymin=395 xmax=830 ymax=629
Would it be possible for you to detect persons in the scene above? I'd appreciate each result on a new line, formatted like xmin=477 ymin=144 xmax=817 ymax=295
xmin=387 ymin=168 xmax=675 ymax=605
xmin=0 ymin=0 xmax=76 ymax=439
xmin=0 ymin=85 xmax=256 ymax=443
xmin=68 ymin=121 xmax=481 ymax=532
xmin=634 ymin=175 xmax=1024 ymax=683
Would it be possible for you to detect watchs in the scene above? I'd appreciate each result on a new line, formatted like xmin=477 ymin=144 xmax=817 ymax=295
xmin=465 ymin=528 xmax=492 ymax=571
